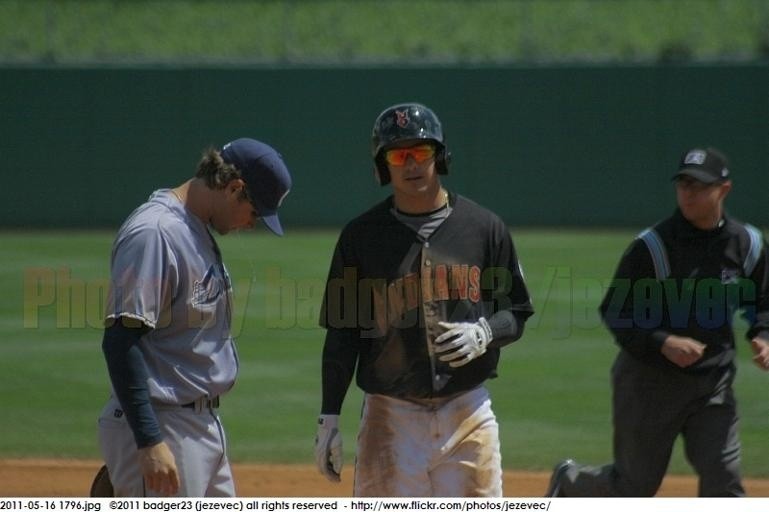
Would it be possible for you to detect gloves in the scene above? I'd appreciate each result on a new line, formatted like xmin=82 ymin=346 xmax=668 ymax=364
xmin=434 ymin=317 xmax=494 ymax=368
xmin=314 ymin=415 xmax=343 ymax=483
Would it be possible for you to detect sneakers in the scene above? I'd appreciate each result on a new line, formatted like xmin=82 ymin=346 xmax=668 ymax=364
xmin=544 ymin=457 xmax=579 ymax=497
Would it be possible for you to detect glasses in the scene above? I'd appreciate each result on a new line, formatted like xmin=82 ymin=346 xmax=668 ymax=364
xmin=675 ymin=178 xmax=714 ymax=192
xmin=382 ymin=142 xmax=437 ymax=167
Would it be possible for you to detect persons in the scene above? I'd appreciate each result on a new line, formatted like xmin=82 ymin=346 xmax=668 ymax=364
xmin=543 ymin=145 xmax=769 ymax=497
xmin=99 ymin=138 xmax=292 ymax=497
xmin=313 ymin=103 xmax=534 ymax=497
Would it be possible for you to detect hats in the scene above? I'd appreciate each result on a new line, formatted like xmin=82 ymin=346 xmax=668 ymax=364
xmin=220 ymin=138 xmax=292 ymax=237
xmin=671 ymin=147 xmax=731 ymax=185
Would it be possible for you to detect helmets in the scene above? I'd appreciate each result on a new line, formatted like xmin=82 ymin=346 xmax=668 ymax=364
xmin=371 ymin=102 xmax=451 ymax=186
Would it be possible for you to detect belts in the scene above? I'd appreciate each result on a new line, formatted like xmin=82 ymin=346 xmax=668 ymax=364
xmin=181 ymin=395 xmax=219 ymax=408
